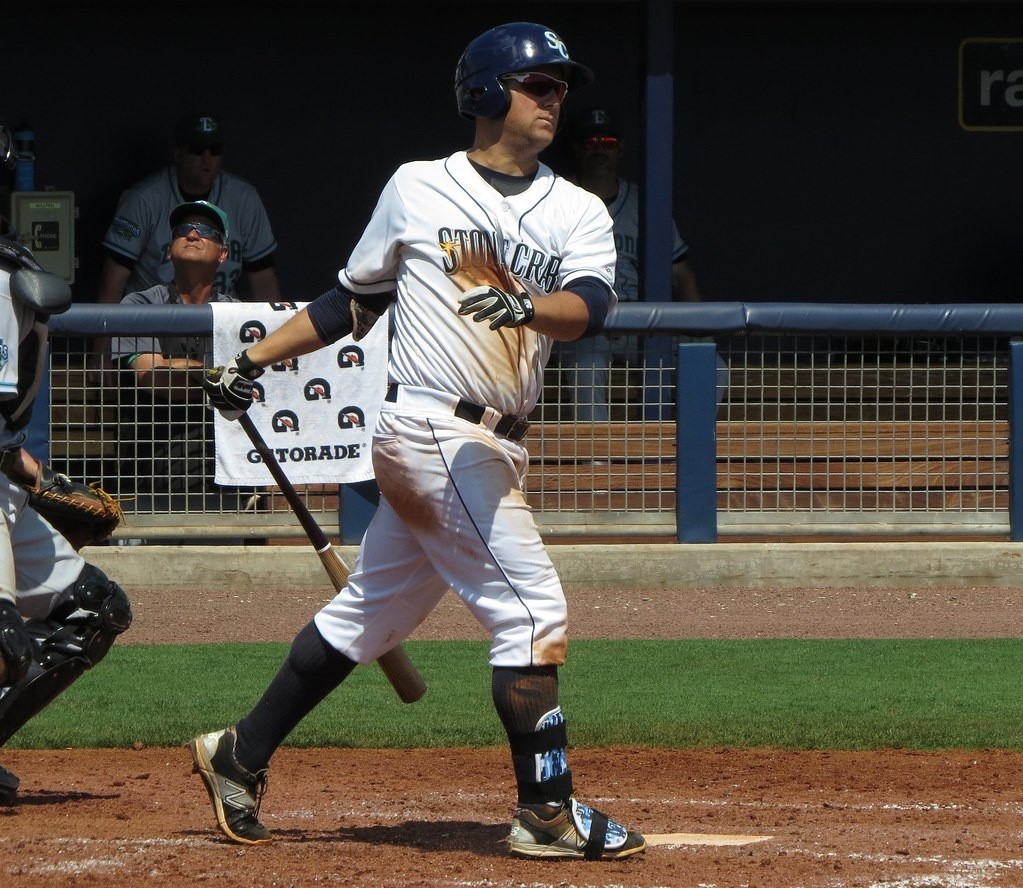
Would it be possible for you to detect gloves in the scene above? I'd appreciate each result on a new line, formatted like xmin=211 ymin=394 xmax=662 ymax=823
xmin=201 ymin=349 xmax=265 ymax=421
xmin=457 ymin=285 xmax=535 ymax=330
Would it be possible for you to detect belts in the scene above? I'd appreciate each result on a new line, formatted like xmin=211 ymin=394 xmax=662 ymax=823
xmin=385 ymin=383 xmax=531 ymax=443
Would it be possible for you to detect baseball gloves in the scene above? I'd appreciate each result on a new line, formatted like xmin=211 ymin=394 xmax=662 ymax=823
xmin=22 ymin=457 xmax=137 ymax=557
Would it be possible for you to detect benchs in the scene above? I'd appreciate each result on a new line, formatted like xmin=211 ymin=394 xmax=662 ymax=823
xmin=51 ymin=346 xmax=1012 ymax=545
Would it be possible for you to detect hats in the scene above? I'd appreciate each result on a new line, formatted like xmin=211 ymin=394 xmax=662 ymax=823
xmin=173 ymin=111 xmax=226 ymax=146
xmin=575 ymin=108 xmax=622 ymax=139
xmin=170 ymin=200 xmax=231 ymax=239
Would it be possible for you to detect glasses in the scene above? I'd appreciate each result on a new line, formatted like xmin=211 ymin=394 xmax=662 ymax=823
xmin=172 ymin=222 xmax=227 ymax=245
xmin=187 ymin=144 xmax=224 ymax=157
xmin=501 ymin=72 xmax=568 ymax=103
xmin=580 ymin=136 xmax=618 ymax=151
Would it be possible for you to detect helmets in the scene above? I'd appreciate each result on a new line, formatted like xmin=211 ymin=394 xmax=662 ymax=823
xmin=455 ymin=22 xmax=593 ymax=122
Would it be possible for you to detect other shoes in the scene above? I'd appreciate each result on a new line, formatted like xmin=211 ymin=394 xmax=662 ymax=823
xmin=0 ymin=766 xmax=20 ymax=795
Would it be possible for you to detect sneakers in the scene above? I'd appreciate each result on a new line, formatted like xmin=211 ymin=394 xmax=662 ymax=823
xmin=507 ymin=799 xmax=648 ymax=857
xmin=184 ymin=725 xmax=273 ymax=846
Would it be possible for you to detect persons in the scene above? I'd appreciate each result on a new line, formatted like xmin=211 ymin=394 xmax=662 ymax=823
xmin=1 ymin=125 xmax=134 ymax=796
xmin=91 ymin=114 xmax=282 ymax=390
xmin=109 ymin=199 xmax=245 ymax=545
xmin=557 ymin=106 xmax=728 ymax=420
xmin=190 ymin=23 xmax=645 ymax=862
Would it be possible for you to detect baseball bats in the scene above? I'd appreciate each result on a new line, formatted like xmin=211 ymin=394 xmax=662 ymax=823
xmin=209 ymin=363 xmax=430 ymax=707
